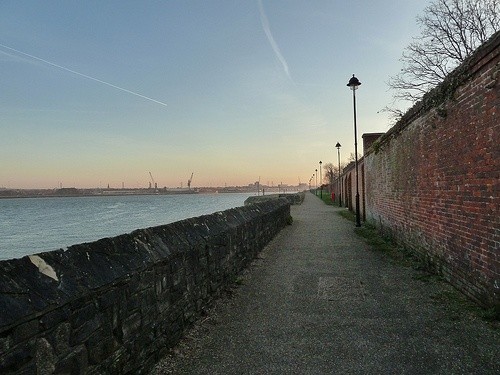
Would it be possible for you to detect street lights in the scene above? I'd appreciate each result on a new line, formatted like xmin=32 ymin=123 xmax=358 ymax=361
xmin=347 ymin=73 xmax=362 ymax=229
xmin=335 ymin=142 xmax=342 ymax=207
xmin=308 ymin=161 xmax=323 ymax=200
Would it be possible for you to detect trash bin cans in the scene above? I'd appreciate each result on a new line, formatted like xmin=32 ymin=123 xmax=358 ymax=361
xmin=331 ymin=191 xmax=335 ymax=199
